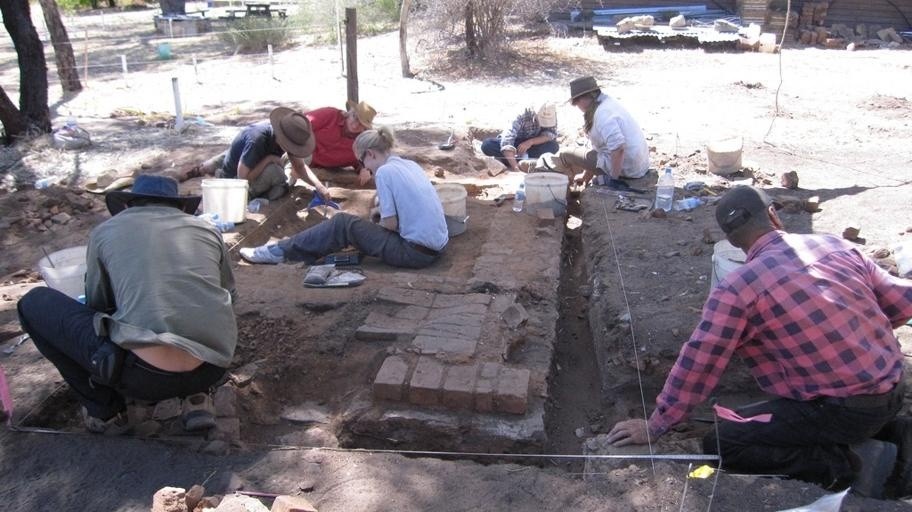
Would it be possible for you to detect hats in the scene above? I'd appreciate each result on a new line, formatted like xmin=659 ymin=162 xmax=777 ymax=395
xmin=716 ymin=184 xmax=784 ymax=233
xmin=105 ymin=175 xmax=202 ymax=214
xmin=347 ymin=98 xmax=376 ymax=129
xmin=270 ymin=107 xmax=316 ymax=157
xmin=565 ymin=76 xmax=602 ymax=102
xmin=536 ymin=99 xmax=556 ymax=128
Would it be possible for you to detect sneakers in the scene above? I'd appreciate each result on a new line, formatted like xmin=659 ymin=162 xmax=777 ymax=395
xmin=519 ymin=159 xmax=540 ymax=172
xmin=848 ymin=439 xmax=897 ymax=499
xmin=881 ymin=410 xmax=912 ymax=498
xmin=180 ymin=393 xmax=217 ymax=431
xmin=239 ymin=245 xmax=285 ymax=264
xmin=82 ymin=405 xmax=127 ymax=430
xmin=268 ymin=182 xmax=288 ymax=200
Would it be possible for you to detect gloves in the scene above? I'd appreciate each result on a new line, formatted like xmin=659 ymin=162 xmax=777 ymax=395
xmin=593 ymin=174 xmax=613 ymax=186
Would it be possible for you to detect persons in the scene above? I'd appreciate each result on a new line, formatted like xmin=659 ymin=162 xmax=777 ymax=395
xmin=240 ymin=126 xmax=450 ymax=269
xmin=216 ymin=106 xmax=331 ymax=215
xmin=518 ymin=76 xmax=649 ymax=187
xmin=607 ymin=184 xmax=912 ymax=498
xmin=481 ymin=100 xmax=559 ymax=172
xmin=179 ymin=99 xmax=377 ymax=186
xmin=17 ymin=174 xmax=238 ymax=436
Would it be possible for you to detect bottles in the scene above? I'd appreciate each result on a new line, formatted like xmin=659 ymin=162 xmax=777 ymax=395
xmin=247 ymin=199 xmax=261 ymax=213
xmin=673 ymin=197 xmax=705 ymax=212
xmin=655 ymin=168 xmax=675 ymax=212
xmin=198 ymin=213 xmax=218 ymax=223
xmin=213 ymin=222 xmax=235 ymax=232
xmin=512 ymin=183 xmax=525 ymax=212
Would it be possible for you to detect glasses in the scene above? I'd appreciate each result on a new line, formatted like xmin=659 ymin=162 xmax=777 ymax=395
xmin=352 ymin=112 xmax=366 ymax=130
xmin=359 ymin=149 xmax=367 ymax=167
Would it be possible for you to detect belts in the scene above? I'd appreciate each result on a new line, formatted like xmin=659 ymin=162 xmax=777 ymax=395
xmin=820 ymin=373 xmax=903 ymax=408
xmin=133 ymin=363 xmax=177 ymax=377
xmin=409 ymin=242 xmax=442 ymax=257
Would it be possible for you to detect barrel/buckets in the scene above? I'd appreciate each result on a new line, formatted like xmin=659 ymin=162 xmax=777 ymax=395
xmin=704 ymin=136 xmax=743 ymax=175
xmin=40 ymin=245 xmax=91 ymax=306
xmin=524 ymin=173 xmax=569 ymax=218
xmin=436 ymin=185 xmax=470 ymax=237
xmin=201 ymin=178 xmax=249 ymax=224
